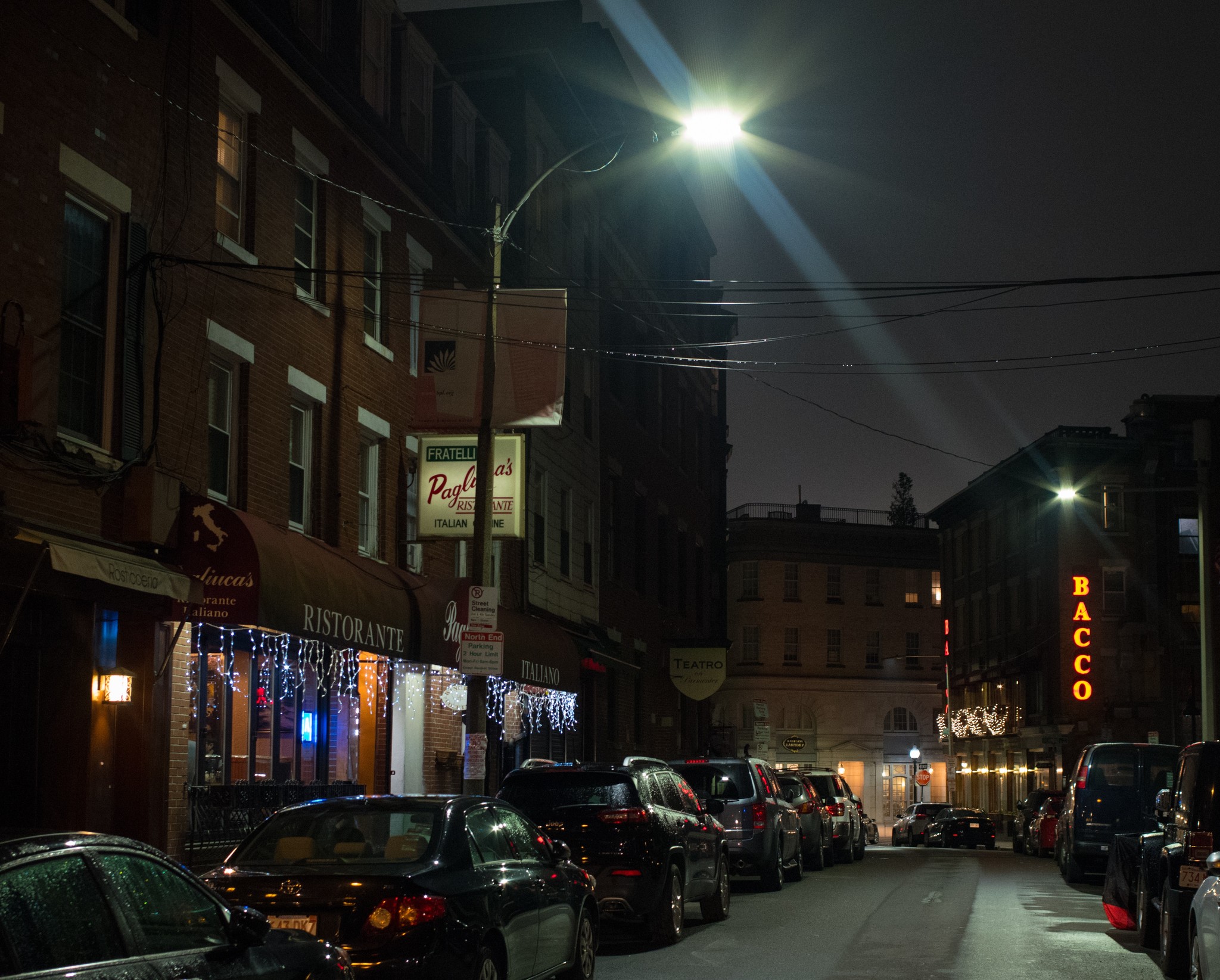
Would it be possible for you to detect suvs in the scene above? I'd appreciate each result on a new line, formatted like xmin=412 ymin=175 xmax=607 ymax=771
xmin=773 ymin=767 xmax=836 ymax=872
xmin=782 ymin=766 xmax=879 ymax=863
xmin=660 ymin=754 xmax=804 ymax=890
xmin=891 ymin=801 xmax=954 ymax=847
xmin=492 ymin=755 xmax=733 ymax=949
xmin=1134 ymin=740 xmax=1219 ymax=977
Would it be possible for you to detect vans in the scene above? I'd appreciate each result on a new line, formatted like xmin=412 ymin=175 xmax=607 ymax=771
xmin=1050 ymin=743 xmax=1181 ymax=886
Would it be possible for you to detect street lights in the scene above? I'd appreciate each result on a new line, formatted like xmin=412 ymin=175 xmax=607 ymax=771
xmin=892 ymin=653 xmax=957 ymax=805
xmin=458 ymin=98 xmax=742 ymax=796
xmin=1043 ymin=466 xmax=1215 ymax=747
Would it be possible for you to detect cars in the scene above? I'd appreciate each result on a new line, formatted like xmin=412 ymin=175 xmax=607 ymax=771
xmin=1008 ymin=788 xmax=1052 ymax=853
xmin=194 ymin=791 xmax=604 ymax=980
xmin=923 ymin=808 xmax=997 ymax=850
xmin=1188 ymin=853 xmax=1220 ymax=980
xmin=0 ymin=831 xmax=361 ymax=980
xmin=1022 ymin=795 xmax=1065 ymax=859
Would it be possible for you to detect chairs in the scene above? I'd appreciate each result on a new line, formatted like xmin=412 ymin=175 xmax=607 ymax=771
xmin=274 ymin=835 xmax=428 ymax=862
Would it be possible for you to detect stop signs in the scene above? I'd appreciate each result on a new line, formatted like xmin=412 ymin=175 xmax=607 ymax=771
xmin=916 ymin=770 xmax=931 ymax=785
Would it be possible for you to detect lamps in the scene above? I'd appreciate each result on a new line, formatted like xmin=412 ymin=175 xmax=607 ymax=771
xmin=98 ymin=665 xmax=137 ymax=707
